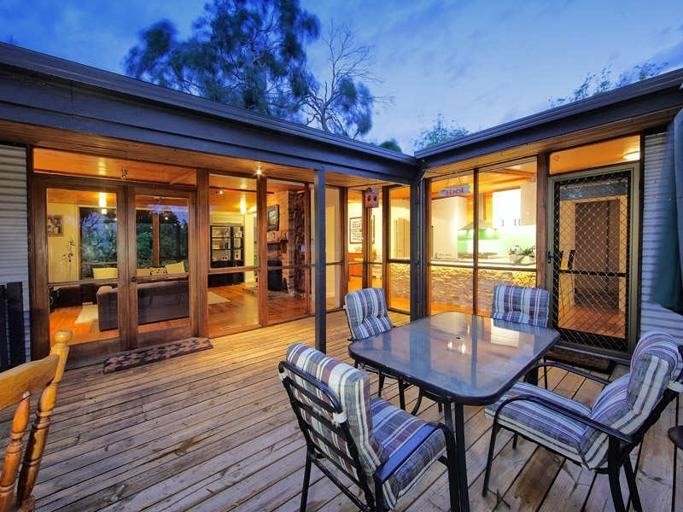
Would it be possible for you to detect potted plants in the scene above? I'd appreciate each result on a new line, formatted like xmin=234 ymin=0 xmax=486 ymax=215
xmin=509 ymin=245 xmax=536 ymax=265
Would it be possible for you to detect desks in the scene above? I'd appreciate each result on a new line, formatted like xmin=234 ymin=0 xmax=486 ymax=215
xmin=348 ymin=310 xmax=561 ymax=511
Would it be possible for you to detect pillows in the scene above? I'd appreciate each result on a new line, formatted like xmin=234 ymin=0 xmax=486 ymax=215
xmin=92 ymin=260 xmax=185 ymax=280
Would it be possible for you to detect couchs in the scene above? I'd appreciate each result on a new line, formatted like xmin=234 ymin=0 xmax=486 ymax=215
xmin=97 ymin=279 xmax=189 ymax=331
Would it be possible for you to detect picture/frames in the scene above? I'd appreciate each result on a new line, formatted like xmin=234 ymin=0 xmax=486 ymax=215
xmin=266 ymin=204 xmax=279 ymax=231
xmin=349 ymin=215 xmax=375 ymax=245
xmin=47 ymin=215 xmax=63 ymax=238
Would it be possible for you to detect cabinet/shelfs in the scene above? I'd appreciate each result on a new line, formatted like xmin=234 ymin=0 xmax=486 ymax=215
xmin=208 ymin=226 xmax=243 ymax=287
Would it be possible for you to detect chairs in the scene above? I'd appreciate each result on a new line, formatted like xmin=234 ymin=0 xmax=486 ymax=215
xmin=482 ymin=330 xmax=682 ymax=511
xmin=490 ymin=283 xmax=553 ymax=387
xmin=343 ymin=287 xmax=413 ymax=411
xmin=0 ymin=330 xmax=71 ymax=512
xmin=277 ymin=341 xmax=462 ymax=511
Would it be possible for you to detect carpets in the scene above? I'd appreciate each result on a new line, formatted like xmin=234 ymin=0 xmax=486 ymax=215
xmin=103 ymin=337 xmax=213 ymax=374
xmin=208 ymin=291 xmax=231 ymax=305
xmin=74 ymin=304 xmax=98 ymax=325
